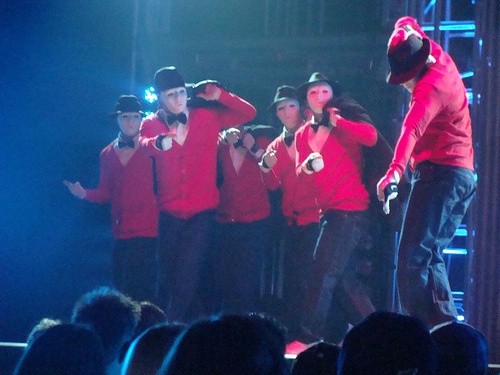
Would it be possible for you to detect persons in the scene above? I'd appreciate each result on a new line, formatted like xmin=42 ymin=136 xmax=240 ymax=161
xmin=62 ymin=65 xmax=378 ymax=360
xmin=376 ymin=16 xmax=478 ymax=329
xmin=13 ymin=285 xmax=489 ymax=375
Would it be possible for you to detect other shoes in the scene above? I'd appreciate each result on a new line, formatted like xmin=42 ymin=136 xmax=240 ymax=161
xmin=285 ymin=340 xmax=310 ymax=355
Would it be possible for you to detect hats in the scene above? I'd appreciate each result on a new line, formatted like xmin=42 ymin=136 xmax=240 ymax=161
xmin=268 ymin=85 xmax=301 ymax=113
xmin=386 ymin=34 xmax=431 ymax=85
xmin=296 ymin=72 xmax=345 ymax=101
xmin=106 ymin=94 xmax=147 ymax=118
xmin=152 ymin=66 xmax=185 ymax=94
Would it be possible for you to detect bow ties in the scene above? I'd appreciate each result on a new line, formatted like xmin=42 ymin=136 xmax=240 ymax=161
xmin=118 ymin=141 xmax=136 ymax=148
xmin=310 ymin=116 xmax=329 ymax=133
xmin=167 ymin=112 xmax=187 ymax=126
xmin=233 ymin=139 xmax=246 ymax=148
xmin=284 ymin=132 xmax=296 ymax=148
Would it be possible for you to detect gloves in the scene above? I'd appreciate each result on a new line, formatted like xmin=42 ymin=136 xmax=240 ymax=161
xmin=300 ymin=152 xmax=324 ymax=175
xmin=399 ymin=25 xmax=424 ymax=40
xmin=257 ymin=150 xmax=277 ymax=173
xmin=242 ymin=133 xmax=264 ymax=159
xmin=219 ymin=128 xmax=240 ymax=145
xmin=377 ymin=170 xmax=401 ymax=214
xmin=151 ymin=133 xmax=178 ymax=152
xmin=63 ymin=181 xmax=86 ymax=200
